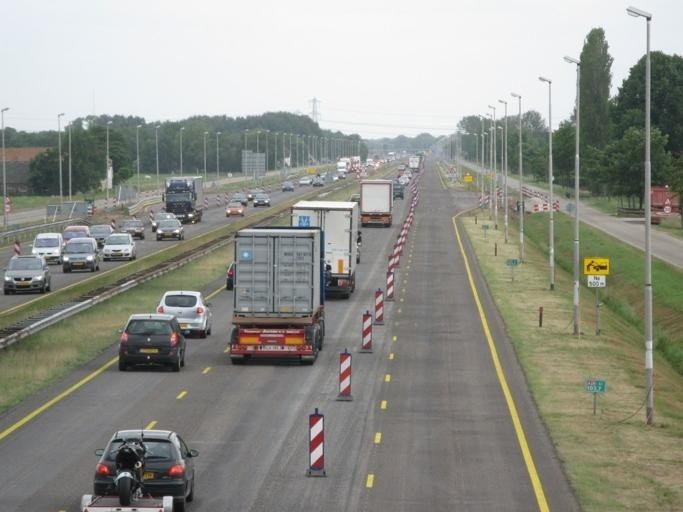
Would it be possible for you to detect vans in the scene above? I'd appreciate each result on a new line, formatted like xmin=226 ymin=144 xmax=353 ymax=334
xmin=29 ymin=232 xmax=64 ymax=265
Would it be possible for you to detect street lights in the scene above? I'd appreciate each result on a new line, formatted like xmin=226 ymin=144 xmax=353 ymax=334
xmin=626 ymin=5 xmax=653 ymax=426
xmin=156 ymin=125 xmax=161 ymax=196
xmin=538 ymin=76 xmax=555 ymax=290
xmin=180 ymin=127 xmax=185 ymax=177
xmin=244 ymin=129 xmax=361 ymax=191
xmin=433 ymin=98 xmax=508 ymax=244
xmin=564 ymin=56 xmax=581 ymax=340
xmin=216 ymin=132 xmax=222 ymax=192
xmin=136 ymin=124 xmax=142 ymax=203
xmin=204 ymin=132 xmax=208 ymax=192
xmin=107 ymin=121 xmax=113 ymax=214
xmin=58 ymin=112 xmax=64 ymax=217
xmin=511 ymin=92 xmax=524 ymax=263
xmin=1 ymin=107 xmax=9 ymax=232
xmin=69 ymin=119 xmax=77 ymax=211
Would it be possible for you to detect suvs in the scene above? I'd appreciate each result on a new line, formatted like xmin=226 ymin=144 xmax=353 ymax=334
xmin=61 ymin=237 xmax=101 ymax=273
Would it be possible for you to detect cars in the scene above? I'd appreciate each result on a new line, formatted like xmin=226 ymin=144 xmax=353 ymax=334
xmin=102 ymin=233 xmax=137 ymax=262
xmin=94 ymin=422 xmax=200 ymax=512
xmin=3 ymin=254 xmax=52 ymax=295
xmin=119 ymin=219 xmax=147 ymax=240
xmin=156 ymin=287 xmax=214 ymax=338
xmin=225 ymin=146 xmax=436 ymax=218
xmin=118 ymin=312 xmax=191 ymax=372
xmin=61 ymin=225 xmax=90 ymax=248
xmin=226 ymin=262 xmax=234 ymax=290
xmin=156 ymin=218 xmax=184 ymax=240
xmin=88 ymin=224 xmax=115 ymax=247
xmin=152 ymin=212 xmax=176 ymax=232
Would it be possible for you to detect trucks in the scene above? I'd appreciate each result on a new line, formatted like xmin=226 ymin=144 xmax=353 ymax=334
xmin=229 ymin=226 xmax=331 ymax=365
xmin=291 ymin=200 xmax=362 ymax=299
xmin=162 ymin=176 xmax=204 ymax=224
xmin=359 ymin=179 xmax=393 ymax=227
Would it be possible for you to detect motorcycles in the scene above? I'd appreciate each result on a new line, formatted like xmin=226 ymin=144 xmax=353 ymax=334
xmin=110 ymin=436 xmax=153 ymax=506
